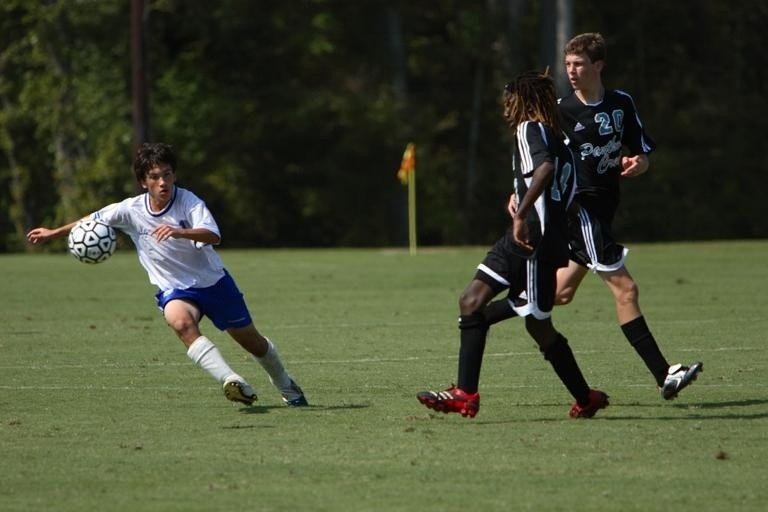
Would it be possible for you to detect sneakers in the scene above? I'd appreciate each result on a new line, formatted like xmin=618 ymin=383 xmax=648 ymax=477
xmin=270 ymin=376 xmax=307 ymax=406
xmin=569 ymin=389 xmax=610 ymax=419
xmin=416 ymin=386 xmax=480 ymax=418
xmin=222 ymin=379 xmax=257 ymax=407
xmin=658 ymin=363 xmax=703 ymax=400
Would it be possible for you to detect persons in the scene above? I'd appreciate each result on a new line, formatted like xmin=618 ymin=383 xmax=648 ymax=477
xmin=457 ymin=30 xmax=704 ymax=400
xmin=25 ymin=140 xmax=312 ymax=410
xmin=414 ymin=64 xmax=613 ymax=420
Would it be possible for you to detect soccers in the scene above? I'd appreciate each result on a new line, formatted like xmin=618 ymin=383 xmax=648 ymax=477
xmin=69 ymin=217 xmax=117 ymax=264
